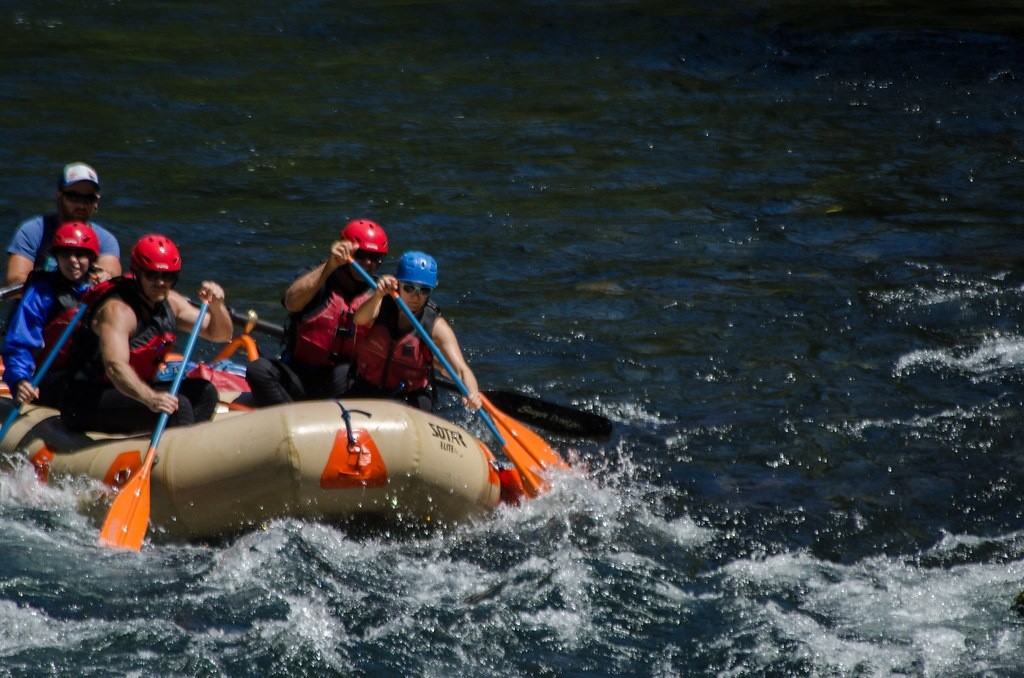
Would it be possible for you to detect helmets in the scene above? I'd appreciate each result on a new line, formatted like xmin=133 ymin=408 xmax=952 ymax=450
xmin=396 ymin=251 xmax=438 ymax=289
xmin=130 ymin=233 xmax=182 ymax=274
xmin=49 ymin=220 xmax=100 ymax=262
xmin=342 ymin=218 xmax=389 ymax=255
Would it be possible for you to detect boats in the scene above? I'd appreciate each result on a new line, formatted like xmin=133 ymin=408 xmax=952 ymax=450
xmin=0 ymin=355 xmax=504 ymax=547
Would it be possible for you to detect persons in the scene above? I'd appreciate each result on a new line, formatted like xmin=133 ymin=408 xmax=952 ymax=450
xmin=338 ymin=251 xmax=482 ymax=414
xmin=245 ymin=216 xmax=387 ymax=407
xmin=5 ymin=162 xmax=124 ymax=333
xmin=53 ymin=232 xmax=234 ymax=433
xmin=2 ymin=221 xmax=101 ymax=408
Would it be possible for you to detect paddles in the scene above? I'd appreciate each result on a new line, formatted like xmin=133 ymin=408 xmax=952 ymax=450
xmin=345 ymin=254 xmax=583 ymax=516
xmin=99 ymin=300 xmax=209 ymax=554
xmin=187 ymin=296 xmax=617 ymax=445
xmin=0 ymin=278 xmax=99 ymax=446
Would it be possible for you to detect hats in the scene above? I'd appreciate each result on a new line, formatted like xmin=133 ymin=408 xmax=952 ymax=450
xmin=58 ymin=161 xmax=101 ymax=193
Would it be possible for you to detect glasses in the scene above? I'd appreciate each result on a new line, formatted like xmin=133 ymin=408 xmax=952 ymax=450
xmin=60 ymin=190 xmax=101 ymax=205
xmin=355 ymin=250 xmax=384 ymax=265
xmin=399 ymin=283 xmax=433 ymax=298
xmin=141 ymin=269 xmax=178 ymax=282
xmin=55 ymin=247 xmax=89 ymax=258
